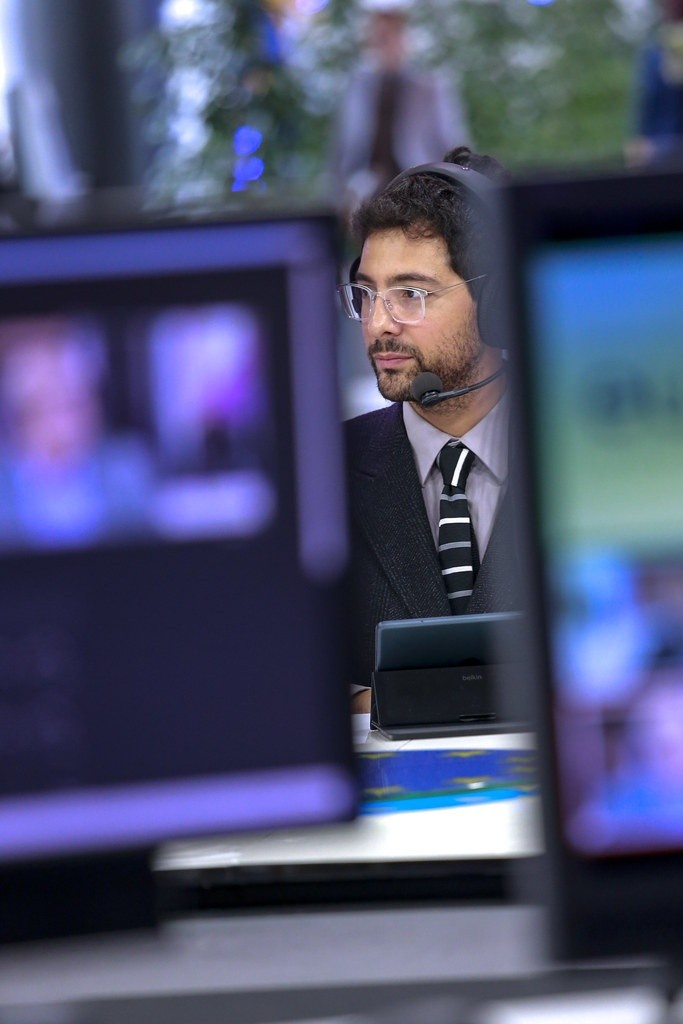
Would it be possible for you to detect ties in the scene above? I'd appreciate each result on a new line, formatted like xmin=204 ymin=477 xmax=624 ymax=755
xmin=433 ymin=444 xmax=480 ymax=617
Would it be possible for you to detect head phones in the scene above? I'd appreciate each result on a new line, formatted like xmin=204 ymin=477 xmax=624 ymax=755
xmin=348 ymin=162 xmax=514 ymax=350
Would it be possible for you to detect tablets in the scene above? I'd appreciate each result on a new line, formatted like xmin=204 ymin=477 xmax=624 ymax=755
xmin=375 ymin=611 xmax=521 ymax=670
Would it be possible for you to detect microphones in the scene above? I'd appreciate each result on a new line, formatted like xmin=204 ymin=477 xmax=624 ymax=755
xmin=412 ymin=362 xmax=506 ymax=407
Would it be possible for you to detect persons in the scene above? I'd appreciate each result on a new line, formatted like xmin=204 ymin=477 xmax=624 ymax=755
xmin=335 ymin=144 xmax=514 ymax=716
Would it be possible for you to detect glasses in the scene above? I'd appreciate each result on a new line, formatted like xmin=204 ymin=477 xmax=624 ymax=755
xmin=336 ymin=272 xmax=487 ymax=325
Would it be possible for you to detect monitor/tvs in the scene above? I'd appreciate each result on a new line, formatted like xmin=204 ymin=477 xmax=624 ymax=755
xmin=500 ymin=168 xmax=683 ymax=965
xmin=0 ymin=206 xmax=363 ymax=948
